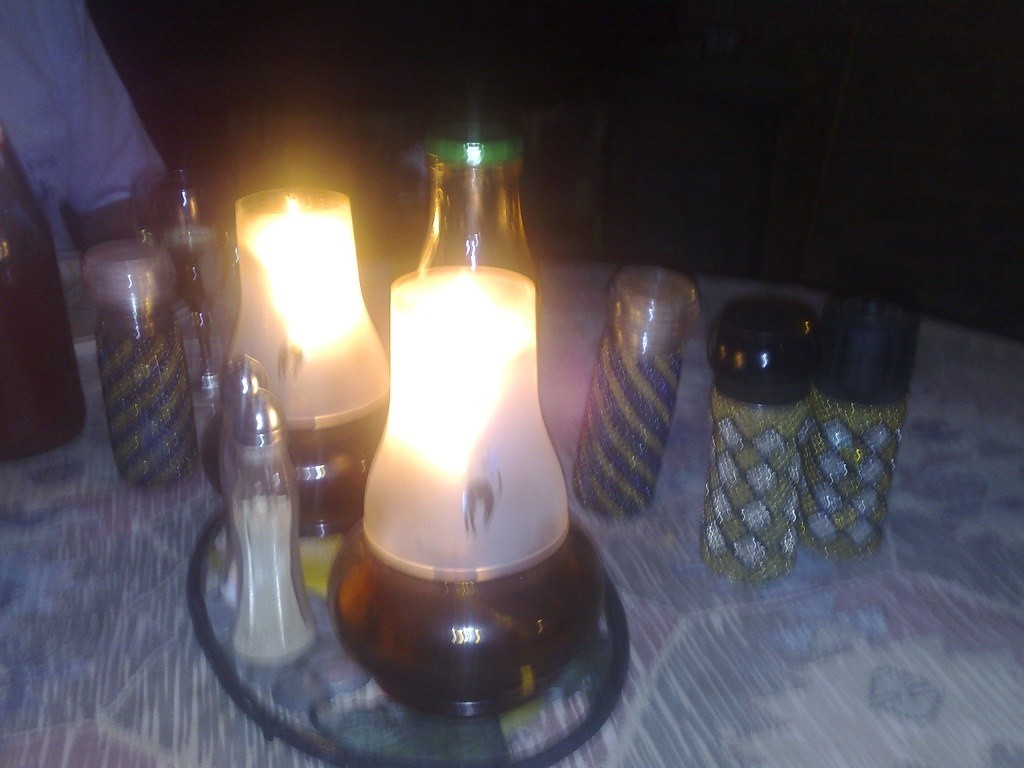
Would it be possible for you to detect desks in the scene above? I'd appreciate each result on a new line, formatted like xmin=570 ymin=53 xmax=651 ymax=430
xmin=0 ymin=264 xmax=1023 ymax=768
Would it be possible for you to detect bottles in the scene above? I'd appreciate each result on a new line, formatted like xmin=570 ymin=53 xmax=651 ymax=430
xmin=219 ymin=354 xmax=319 ymax=668
xmin=572 ymin=266 xmax=699 ymax=518
xmin=0 ymin=122 xmax=87 ymax=463
xmin=84 ymin=241 xmax=201 ymax=488
xmin=796 ymin=298 xmax=918 ymax=565
xmin=407 ymin=123 xmax=543 ymax=339
xmin=164 ymin=167 xmax=214 ymax=370
xmin=699 ymin=299 xmax=816 ymax=582
